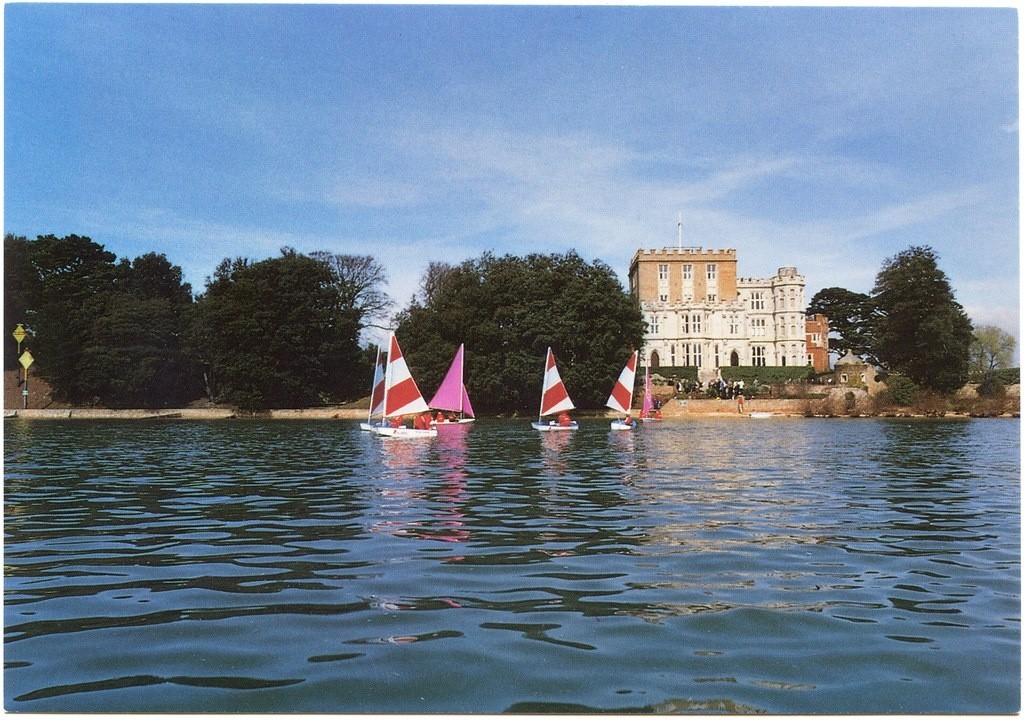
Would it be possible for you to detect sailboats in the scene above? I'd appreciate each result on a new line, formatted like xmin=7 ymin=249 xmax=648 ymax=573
xmin=359 ymin=330 xmax=439 ymax=438
xmin=604 ymin=349 xmax=637 ymax=431
xmin=638 ymin=357 xmax=662 ymax=422
xmin=529 ymin=347 xmax=579 ymax=430
xmin=427 ymin=343 xmax=476 ymax=424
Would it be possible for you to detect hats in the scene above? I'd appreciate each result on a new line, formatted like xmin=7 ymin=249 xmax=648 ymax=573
xmin=563 ymin=414 xmax=566 ymax=416
xmin=626 ymin=414 xmax=629 ymax=417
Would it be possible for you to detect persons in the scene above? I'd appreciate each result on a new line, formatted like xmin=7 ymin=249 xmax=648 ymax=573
xmin=448 ymin=412 xmax=460 ymax=422
xmin=558 ymin=414 xmax=571 ymax=426
xmin=737 ymin=392 xmax=744 ymax=413
xmin=415 ymin=414 xmax=433 ymax=429
xmin=656 ymin=393 xmax=664 ymax=408
xmin=653 ymin=410 xmax=662 ymax=418
xmin=437 ymin=412 xmax=444 ymax=423
xmin=390 ymin=416 xmax=402 ymax=427
xmin=625 ymin=415 xmax=631 ymax=425
xmin=675 ymin=377 xmax=745 ymax=399
